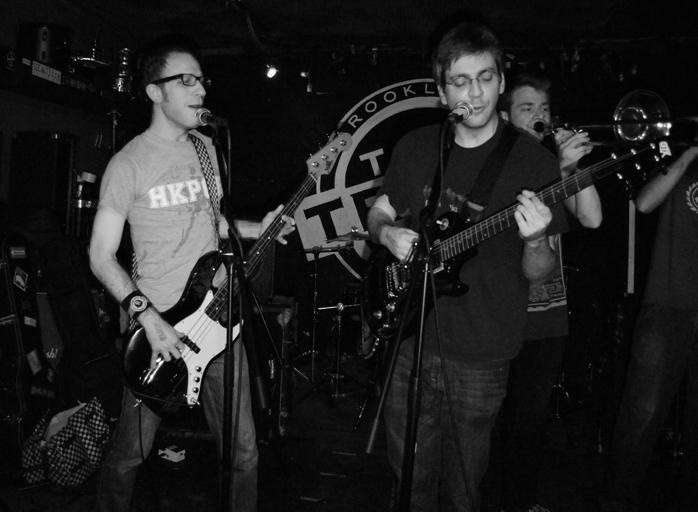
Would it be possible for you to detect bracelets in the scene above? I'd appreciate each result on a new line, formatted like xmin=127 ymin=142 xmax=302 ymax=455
xmin=120 ymin=289 xmax=153 ymax=321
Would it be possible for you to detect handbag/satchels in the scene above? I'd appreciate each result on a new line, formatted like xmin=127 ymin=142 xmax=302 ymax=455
xmin=21 ymin=396 xmax=111 ymax=491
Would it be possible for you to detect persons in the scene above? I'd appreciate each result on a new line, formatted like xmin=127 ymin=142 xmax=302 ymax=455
xmin=364 ymin=24 xmax=569 ymax=506
xmin=86 ymin=46 xmax=298 ymax=510
xmin=602 ymin=120 xmax=697 ymax=504
xmin=482 ymin=77 xmax=604 ymax=486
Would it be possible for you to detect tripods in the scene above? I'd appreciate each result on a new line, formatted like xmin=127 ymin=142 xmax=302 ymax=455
xmin=287 ymin=253 xmax=345 ymax=385
xmin=333 ymin=120 xmax=475 ymax=512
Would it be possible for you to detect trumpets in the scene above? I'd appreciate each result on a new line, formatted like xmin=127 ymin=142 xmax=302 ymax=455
xmin=533 ymin=90 xmax=672 ymax=148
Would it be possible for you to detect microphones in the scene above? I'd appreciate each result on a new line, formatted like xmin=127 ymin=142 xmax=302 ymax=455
xmin=303 ymin=246 xmax=340 ymax=254
xmin=195 ymin=108 xmax=221 ymax=130
xmin=448 ymin=100 xmax=474 ymax=124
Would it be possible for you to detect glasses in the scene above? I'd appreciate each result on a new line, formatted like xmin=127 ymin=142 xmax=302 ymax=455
xmin=151 ymin=74 xmax=211 ymax=87
xmin=446 ymin=70 xmax=501 ymax=91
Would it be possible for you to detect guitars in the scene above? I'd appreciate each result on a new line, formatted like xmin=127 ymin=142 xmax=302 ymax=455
xmin=360 ymin=137 xmax=673 ymax=362
xmin=125 ymin=130 xmax=353 ymax=417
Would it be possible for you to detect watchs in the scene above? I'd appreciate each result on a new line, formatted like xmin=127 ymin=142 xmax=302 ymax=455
xmin=123 ymin=294 xmax=152 ymax=320
